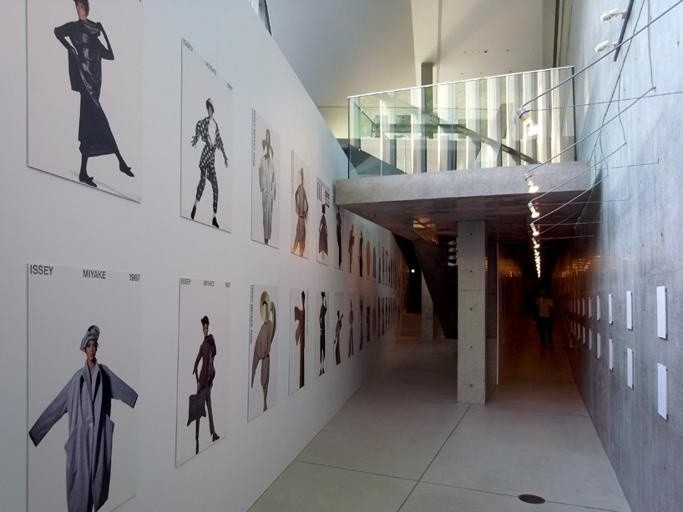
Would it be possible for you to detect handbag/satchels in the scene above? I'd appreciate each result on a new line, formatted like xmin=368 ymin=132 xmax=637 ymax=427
xmin=187 ymin=393 xmax=206 ymax=426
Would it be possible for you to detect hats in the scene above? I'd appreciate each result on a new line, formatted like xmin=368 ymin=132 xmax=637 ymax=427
xmin=201 ymin=316 xmax=209 ymax=326
xmin=80 ymin=325 xmax=100 ymax=350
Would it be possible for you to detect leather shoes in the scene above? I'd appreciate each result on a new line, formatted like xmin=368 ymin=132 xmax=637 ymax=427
xmin=79 ymin=174 xmax=97 ymax=187
xmin=213 ymin=217 xmax=219 ymax=227
xmin=191 ymin=205 xmax=196 ymax=219
xmin=119 ymin=163 xmax=134 ymax=177
xmin=213 ymin=433 xmax=219 ymax=441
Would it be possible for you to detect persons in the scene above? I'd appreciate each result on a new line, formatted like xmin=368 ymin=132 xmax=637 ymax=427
xmin=189 ymin=315 xmax=220 ymax=455
xmin=293 ymin=292 xmax=305 ymax=389
xmin=186 ymin=98 xmax=229 ymax=229
xmin=533 ymin=284 xmax=553 ymax=352
xmin=249 ymin=290 xmax=277 ymax=412
xmin=26 ymin=324 xmax=138 ymax=511
xmin=256 ymin=128 xmax=277 ymax=245
xmin=314 ymin=203 xmax=401 ymax=377
xmin=51 ymin=0 xmax=136 ymax=188
xmin=290 ymin=168 xmax=308 ymax=257
